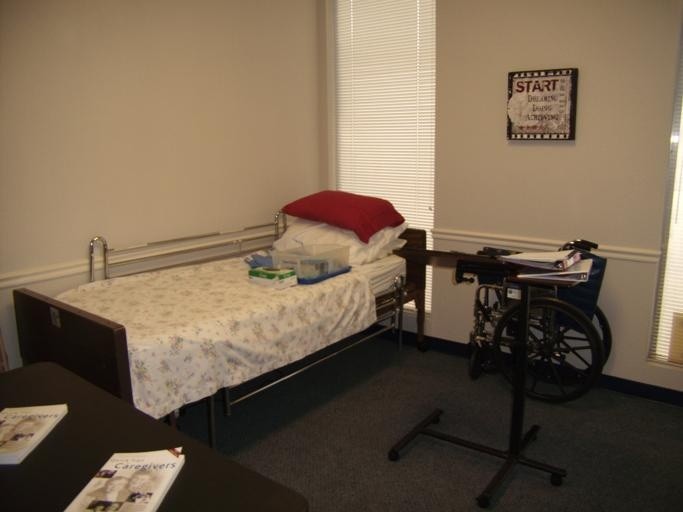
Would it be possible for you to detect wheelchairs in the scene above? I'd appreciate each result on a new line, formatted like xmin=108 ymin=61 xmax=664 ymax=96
xmin=454 ymin=238 xmax=611 ymax=406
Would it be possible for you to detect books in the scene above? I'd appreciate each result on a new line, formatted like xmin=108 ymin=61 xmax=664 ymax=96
xmin=0 ymin=404 xmax=74 ymax=468
xmin=58 ymin=446 xmax=193 ymax=512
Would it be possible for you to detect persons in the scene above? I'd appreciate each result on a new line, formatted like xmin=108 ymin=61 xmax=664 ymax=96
xmin=87 ymin=475 xmax=132 ymax=501
xmin=4 ymin=417 xmax=40 ymax=441
xmin=119 ymin=469 xmax=155 ymax=501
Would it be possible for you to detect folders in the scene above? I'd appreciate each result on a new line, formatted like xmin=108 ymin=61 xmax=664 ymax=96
xmin=500 ymin=250 xmax=593 ymax=282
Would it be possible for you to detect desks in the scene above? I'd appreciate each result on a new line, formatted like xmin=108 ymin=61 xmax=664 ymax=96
xmin=0 ymin=363 xmax=311 ymax=512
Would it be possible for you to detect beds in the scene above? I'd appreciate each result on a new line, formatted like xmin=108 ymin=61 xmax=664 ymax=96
xmin=13 ymin=226 xmax=425 ymax=452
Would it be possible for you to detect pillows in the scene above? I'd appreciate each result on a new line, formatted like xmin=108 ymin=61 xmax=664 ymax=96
xmin=285 ymin=191 xmax=402 ymax=236
xmin=272 ymin=218 xmax=408 ymax=264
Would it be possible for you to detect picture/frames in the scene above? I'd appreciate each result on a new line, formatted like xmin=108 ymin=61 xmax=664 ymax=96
xmin=506 ymin=68 xmax=577 ymax=141
